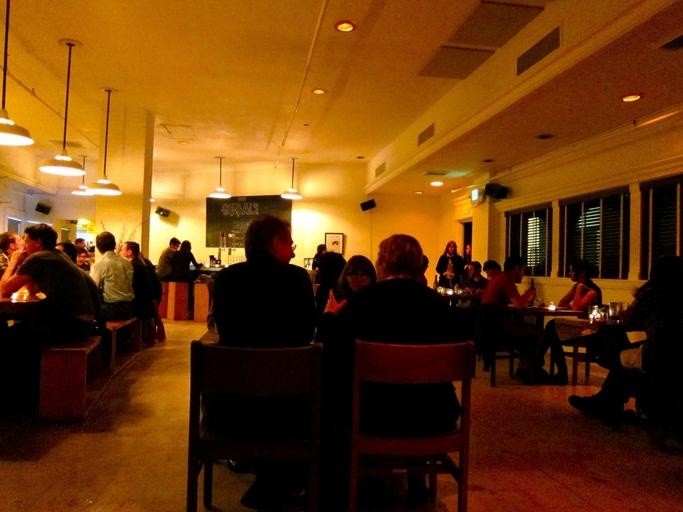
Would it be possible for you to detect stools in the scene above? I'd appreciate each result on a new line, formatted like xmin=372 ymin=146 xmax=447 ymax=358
xmin=194 ymin=283 xmax=209 ymax=322
xmin=167 ymin=282 xmax=188 ymax=320
xmin=158 ymin=282 xmax=167 ymax=319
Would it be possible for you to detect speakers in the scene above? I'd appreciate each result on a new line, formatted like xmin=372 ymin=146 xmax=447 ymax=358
xmin=360 ymin=198 xmax=376 ymax=211
xmin=155 ymin=207 xmax=170 ymax=216
xmin=35 ymin=202 xmax=51 ymax=215
xmin=484 ymin=183 xmax=508 ymax=199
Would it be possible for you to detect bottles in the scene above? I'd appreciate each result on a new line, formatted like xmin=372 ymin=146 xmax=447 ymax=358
xmin=433 ymin=275 xmax=438 ymax=291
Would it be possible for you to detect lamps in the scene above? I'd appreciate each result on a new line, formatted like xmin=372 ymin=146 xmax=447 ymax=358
xmin=281 ymin=159 xmax=302 ymax=199
xmin=72 ymin=155 xmax=87 ymax=196
xmin=0 ymin=0 xmax=34 ymax=146
xmin=208 ymin=156 xmax=231 ymax=199
xmin=85 ymin=88 xmax=122 ymax=195
xmin=40 ymin=38 xmax=86 ymax=176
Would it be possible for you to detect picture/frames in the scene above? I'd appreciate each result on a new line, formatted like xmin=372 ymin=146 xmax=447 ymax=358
xmin=325 ymin=233 xmax=344 ymax=255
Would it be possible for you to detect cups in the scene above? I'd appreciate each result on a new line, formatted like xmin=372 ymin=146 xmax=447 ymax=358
xmin=608 ymin=300 xmax=628 ymax=321
xmin=587 ymin=305 xmax=607 ymax=323
xmin=208 ymin=254 xmax=220 ymax=267
xmin=435 ymin=286 xmax=463 ymax=296
xmin=547 ymin=301 xmax=555 ymax=311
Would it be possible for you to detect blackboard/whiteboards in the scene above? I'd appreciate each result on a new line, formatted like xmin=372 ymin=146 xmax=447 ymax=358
xmin=206 ymin=195 xmax=292 ymax=248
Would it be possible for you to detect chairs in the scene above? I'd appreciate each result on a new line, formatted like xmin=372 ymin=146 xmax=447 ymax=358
xmin=473 ymin=304 xmax=535 ymax=387
xmin=348 ymin=338 xmax=474 ymax=512
xmin=185 ymin=340 xmax=322 ymax=512
xmin=550 ymin=305 xmax=609 ymax=386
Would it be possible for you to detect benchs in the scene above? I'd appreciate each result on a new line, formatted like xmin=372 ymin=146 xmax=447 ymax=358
xmin=106 ymin=317 xmax=141 ymax=377
xmin=39 ymin=329 xmax=112 ymax=420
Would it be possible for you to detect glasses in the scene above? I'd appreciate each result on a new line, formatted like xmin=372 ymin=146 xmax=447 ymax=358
xmin=290 ymin=244 xmax=296 ymax=252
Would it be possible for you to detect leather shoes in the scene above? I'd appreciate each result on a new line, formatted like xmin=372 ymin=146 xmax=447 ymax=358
xmin=568 ymin=395 xmax=624 ymax=431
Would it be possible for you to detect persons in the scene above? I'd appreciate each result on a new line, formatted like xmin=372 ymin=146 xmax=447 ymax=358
xmin=308 ymin=238 xmax=342 ymax=284
xmin=174 ymin=240 xmax=204 ymax=283
xmin=1 ymin=222 xmax=165 ymax=365
xmin=568 ymin=257 xmax=681 ymax=451
xmin=433 ymin=241 xmax=601 ymax=386
xmin=332 ymin=257 xmax=377 ymax=291
xmin=213 ymin=213 xmax=315 ymax=511
xmin=319 ymin=235 xmax=456 ymax=506
xmin=155 ymin=236 xmax=177 ymax=277
